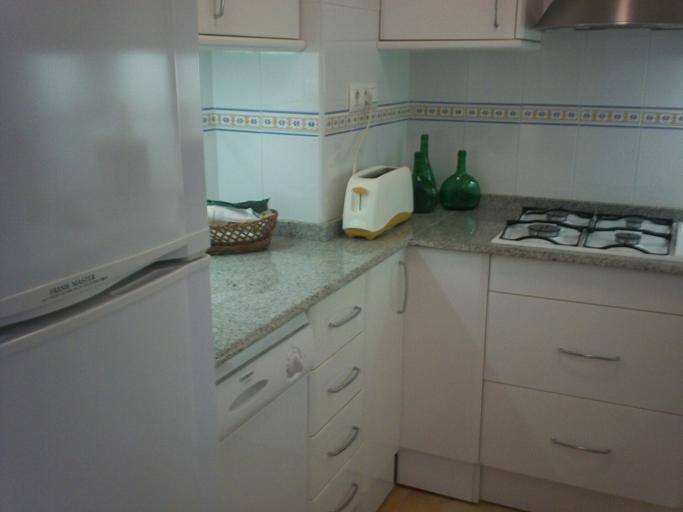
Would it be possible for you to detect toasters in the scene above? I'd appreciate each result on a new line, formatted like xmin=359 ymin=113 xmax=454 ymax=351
xmin=341 ymin=165 xmax=414 ymax=240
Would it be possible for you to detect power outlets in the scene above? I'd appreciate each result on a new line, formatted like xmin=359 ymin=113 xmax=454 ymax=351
xmin=350 ymin=83 xmax=377 ymax=108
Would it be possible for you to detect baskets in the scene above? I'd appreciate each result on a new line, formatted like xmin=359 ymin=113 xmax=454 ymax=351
xmin=206 ymin=209 xmax=277 ymax=255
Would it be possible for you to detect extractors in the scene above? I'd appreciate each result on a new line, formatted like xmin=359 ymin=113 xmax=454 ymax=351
xmin=525 ymin=0 xmax=682 ymax=38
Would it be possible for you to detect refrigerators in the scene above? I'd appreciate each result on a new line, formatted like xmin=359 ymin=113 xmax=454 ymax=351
xmin=1 ymin=2 xmax=218 ymax=510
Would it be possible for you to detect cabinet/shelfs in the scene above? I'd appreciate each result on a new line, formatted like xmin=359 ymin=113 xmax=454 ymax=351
xmin=379 ymin=0 xmax=543 ymax=52
xmin=198 ymin=0 xmax=306 ymax=52
xmin=406 ymin=250 xmax=683 ymax=511
xmin=371 ymin=253 xmax=409 ymax=509
xmin=218 ymin=313 xmax=312 ymax=508
xmin=308 ymin=275 xmax=373 ymax=509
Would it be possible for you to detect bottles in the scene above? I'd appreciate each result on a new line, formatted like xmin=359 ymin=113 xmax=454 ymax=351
xmin=412 ymin=134 xmax=436 ymax=214
xmin=440 ymin=150 xmax=482 ymax=212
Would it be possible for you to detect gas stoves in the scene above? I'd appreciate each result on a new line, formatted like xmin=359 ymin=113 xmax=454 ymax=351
xmin=500 ymin=206 xmax=674 ymax=256
